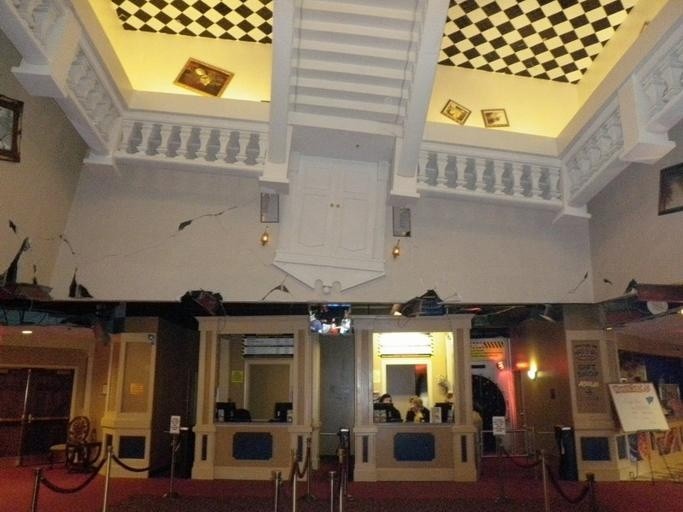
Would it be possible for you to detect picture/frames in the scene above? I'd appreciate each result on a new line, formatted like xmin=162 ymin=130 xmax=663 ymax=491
xmin=658 ymin=163 xmax=683 ymax=215
xmin=173 ymin=57 xmax=235 ymax=98
xmin=0 ymin=94 xmax=25 ymax=162
xmin=440 ymin=99 xmax=471 ymax=125
xmin=481 ymin=109 xmax=509 ymax=127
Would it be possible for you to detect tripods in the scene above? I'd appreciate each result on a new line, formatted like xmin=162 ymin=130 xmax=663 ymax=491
xmin=635 ymin=431 xmax=675 ymax=484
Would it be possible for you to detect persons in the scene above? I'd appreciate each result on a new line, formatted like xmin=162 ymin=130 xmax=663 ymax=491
xmin=406 ymin=395 xmax=430 ymax=424
xmin=379 ymin=394 xmax=401 ymax=422
xmin=308 ymin=309 xmax=350 ymax=335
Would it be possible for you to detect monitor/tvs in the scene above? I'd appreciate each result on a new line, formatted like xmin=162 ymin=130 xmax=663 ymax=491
xmin=275 ymin=403 xmax=292 ymax=422
xmin=308 ymin=303 xmax=353 ymax=337
